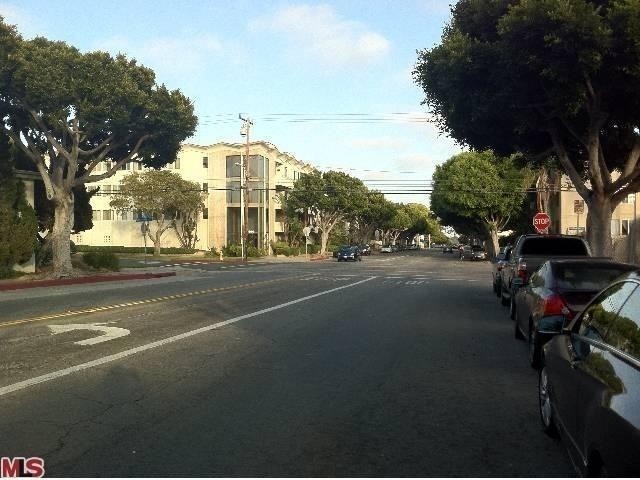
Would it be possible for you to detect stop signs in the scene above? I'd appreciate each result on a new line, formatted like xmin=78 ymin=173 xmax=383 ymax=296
xmin=532 ymin=212 xmax=551 ymax=231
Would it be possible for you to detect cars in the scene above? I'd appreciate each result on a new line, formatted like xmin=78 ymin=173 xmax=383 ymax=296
xmin=493 ymin=243 xmax=512 ymax=296
xmin=538 ymin=267 xmax=639 ymax=478
xmin=432 ymin=242 xmax=487 ymax=263
xmin=509 ymin=256 xmax=639 ymax=370
xmin=333 ymin=241 xmax=418 ymax=262
xmin=499 ymin=234 xmax=592 ymax=306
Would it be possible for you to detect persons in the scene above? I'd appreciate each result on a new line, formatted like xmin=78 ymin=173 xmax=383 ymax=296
xmin=458 ymin=244 xmax=465 ymax=262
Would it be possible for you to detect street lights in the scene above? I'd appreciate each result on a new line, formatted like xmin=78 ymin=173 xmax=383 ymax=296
xmin=234 ymin=122 xmax=252 ymax=261
